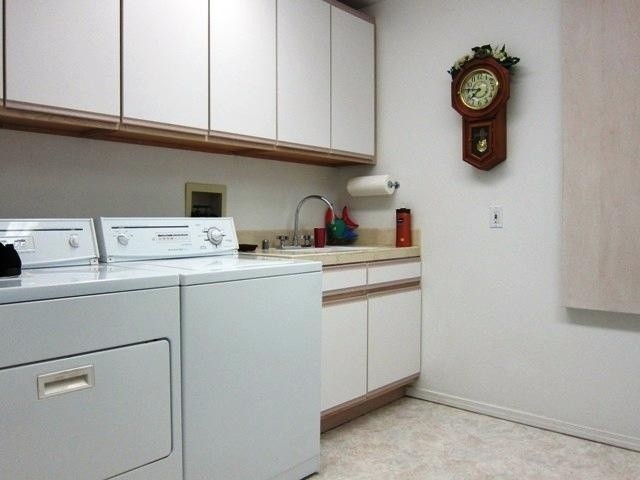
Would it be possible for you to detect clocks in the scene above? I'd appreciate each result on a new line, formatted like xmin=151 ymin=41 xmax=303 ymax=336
xmin=445 ymin=42 xmax=520 ymax=173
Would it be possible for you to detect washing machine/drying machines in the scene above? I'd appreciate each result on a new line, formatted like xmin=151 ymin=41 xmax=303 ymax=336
xmin=0 ymin=217 xmax=183 ymax=480
xmin=100 ymin=217 xmax=322 ymax=480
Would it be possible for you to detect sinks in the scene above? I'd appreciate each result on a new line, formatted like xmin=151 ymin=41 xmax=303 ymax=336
xmin=311 ymin=246 xmax=360 ymax=254
xmin=275 ymin=248 xmax=330 ymax=258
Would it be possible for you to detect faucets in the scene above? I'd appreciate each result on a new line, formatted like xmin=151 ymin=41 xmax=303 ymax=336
xmin=293 ymin=195 xmax=333 ymax=245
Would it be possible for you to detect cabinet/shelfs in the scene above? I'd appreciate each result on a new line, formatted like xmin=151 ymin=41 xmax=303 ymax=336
xmin=115 ymin=1 xmax=211 ymax=152
xmin=1 ymin=0 xmax=123 ymax=138
xmin=274 ymin=2 xmax=332 ymax=165
xmin=206 ymin=1 xmax=278 ymax=158
xmin=320 ymin=245 xmax=422 ymax=439
xmin=329 ymin=2 xmax=379 ymax=166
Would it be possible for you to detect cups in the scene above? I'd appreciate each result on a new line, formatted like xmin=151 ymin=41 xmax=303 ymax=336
xmin=314 ymin=227 xmax=326 ymax=248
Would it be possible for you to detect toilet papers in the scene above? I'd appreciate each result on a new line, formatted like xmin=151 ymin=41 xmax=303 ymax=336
xmin=345 ymin=175 xmax=396 ymax=197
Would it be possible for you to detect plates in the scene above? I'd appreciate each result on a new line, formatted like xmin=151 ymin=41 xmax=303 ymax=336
xmin=238 ymin=244 xmax=258 ymax=251
